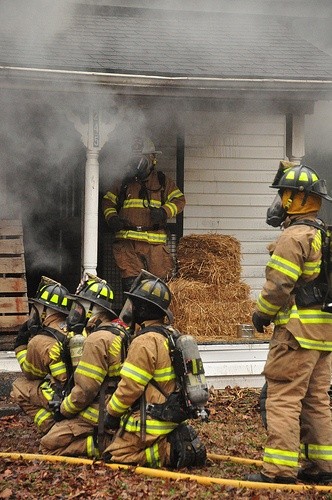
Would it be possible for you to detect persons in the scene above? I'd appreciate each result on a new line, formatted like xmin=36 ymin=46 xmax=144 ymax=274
xmin=102 ymin=271 xmax=208 ymax=467
xmin=39 ymin=273 xmax=131 ymax=455
xmin=102 ymin=136 xmax=188 ymax=304
xmin=10 ymin=275 xmax=85 ymax=431
xmin=245 ymin=160 xmax=332 ymax=484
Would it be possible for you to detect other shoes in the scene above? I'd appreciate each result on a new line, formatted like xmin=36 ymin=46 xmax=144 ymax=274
xmin=242 ymin=471 xmax=295 ymax=483
xmin=185 ymin=425 xmax=206 ymax=469
xmin=166 ymin=423 xmax=195 ymax=469
xmin=296 ymin=470 xmax=332 ymax=483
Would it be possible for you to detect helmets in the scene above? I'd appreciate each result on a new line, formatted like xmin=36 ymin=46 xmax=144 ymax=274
xmin=29 ymin=275 xmax=70 ymax=316
xmin=128 ymin=135 xmax=157 ymax=159
xmin=67 ymin=272 xmax=120 ymax=319
xmin=123 ymin=268 xmax=175 ymax=323
xmin=270 ymin=160 xmax=332 ymax=202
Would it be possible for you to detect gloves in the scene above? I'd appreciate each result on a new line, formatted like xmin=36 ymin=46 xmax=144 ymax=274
xmin=14 ymin=320 xmax=31 ymax=348
xmin=103 ymin=413 xmax=121 ymax=435
xmin=148 ymin=204 xmax=166 ymax=224
xmin=108 ymin=217 xmax=125 ymax=232
xmin=53 ymin=410 xmax=66 ymax=422
xmin=251 ymin=313 xmax=272 ymax=333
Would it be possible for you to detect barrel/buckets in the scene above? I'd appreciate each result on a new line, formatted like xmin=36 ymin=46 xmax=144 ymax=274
xmin=237 ymin=324 xmax=255 ymax=338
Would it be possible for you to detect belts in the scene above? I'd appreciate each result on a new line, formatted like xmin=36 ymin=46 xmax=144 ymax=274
xmin=120 ymin=225 xmax=164 ymax=232
